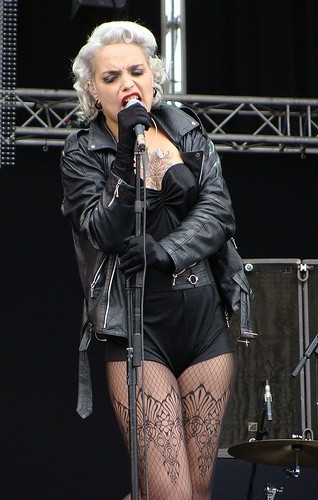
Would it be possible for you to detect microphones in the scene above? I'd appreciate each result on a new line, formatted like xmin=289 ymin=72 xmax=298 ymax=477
xmin=264 ymin=380 xmax=273 ymax=421
xmin=126 ymin=99 xmax=147 ymax=150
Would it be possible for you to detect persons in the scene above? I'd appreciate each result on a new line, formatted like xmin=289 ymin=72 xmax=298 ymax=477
xmin=61 ymin=20 xmax=256 ymax=500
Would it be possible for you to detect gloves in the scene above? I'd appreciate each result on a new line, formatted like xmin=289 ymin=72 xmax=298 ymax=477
xmin=117 ymin=233 xmax=174 ymax=279
xmin=115 ymin=102 xmax=152 ymax=156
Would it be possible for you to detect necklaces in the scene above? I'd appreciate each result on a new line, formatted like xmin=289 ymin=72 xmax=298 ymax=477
xmin=103 ymin=116 xmax=157 ymax=161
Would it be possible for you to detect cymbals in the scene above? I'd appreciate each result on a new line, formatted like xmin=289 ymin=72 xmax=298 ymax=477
xmin=228 ymin=439 xmax=318 ymax=467
